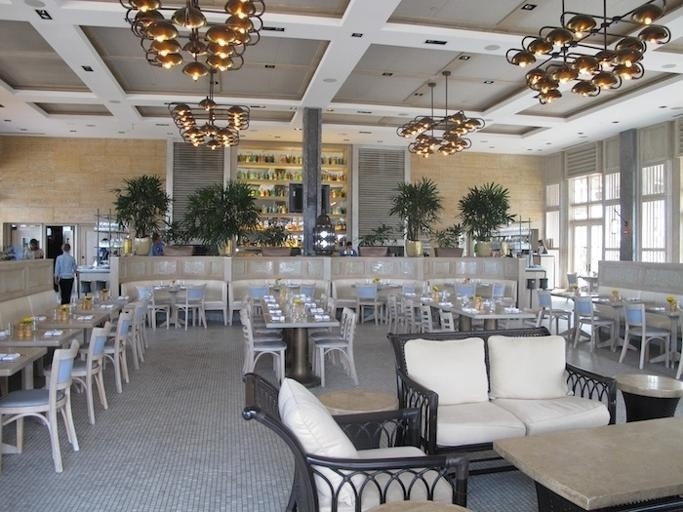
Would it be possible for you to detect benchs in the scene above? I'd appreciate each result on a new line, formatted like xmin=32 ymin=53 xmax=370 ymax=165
xmin=383 ymin=326 xmax=618 ymax=479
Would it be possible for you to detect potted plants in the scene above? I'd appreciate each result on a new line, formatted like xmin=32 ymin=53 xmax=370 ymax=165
xmin=111 ymin=174 xmax=293 ymax=256
xmin=359 ymin=176 xmax=517 ymax=258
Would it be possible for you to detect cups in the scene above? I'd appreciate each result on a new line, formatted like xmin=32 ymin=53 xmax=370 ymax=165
xmin=31 ymin=317 xmax=40 ymax=333
xmin=441 ymin=289 xmax=496 ymax=311
xmin=4 ymin=321 xmax=15 ymax=338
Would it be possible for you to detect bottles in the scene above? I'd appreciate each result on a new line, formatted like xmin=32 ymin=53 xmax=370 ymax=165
xmin=237 ymin=152 xmax=346 ymax=249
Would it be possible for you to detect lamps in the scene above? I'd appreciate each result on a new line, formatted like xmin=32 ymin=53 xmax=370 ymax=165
xmin=396 ymin=71 xmax=485 ymax=158
xmin=312 ymin=208 xmax=337 ymax=256
xmin=164 ymin=74 xmax=250 ymax=151
xmin=505 ymin=0 xmax=672 ymax=104
xmin=119 ymin=0 xmax=266 ymax=83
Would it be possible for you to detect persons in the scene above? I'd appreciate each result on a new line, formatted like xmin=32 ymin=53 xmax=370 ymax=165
xmin=535 ymin=240 xmax=547 ymax=254
xmin=147 ymin=231 xmax=166 ymax=256
xmin=22 ymin=238 xmax=44 ymax=260
xmin=97 ymin=238 xmax=112 ymax=264
xmin=339 ymin=241 xmax=358 ymax=257
xmin=54 ymin=243 xmax=79 ymax=305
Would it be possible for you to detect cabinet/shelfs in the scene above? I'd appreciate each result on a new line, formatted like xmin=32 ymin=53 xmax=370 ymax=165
xmin=237 ymin=148 xmax=348 ymax=247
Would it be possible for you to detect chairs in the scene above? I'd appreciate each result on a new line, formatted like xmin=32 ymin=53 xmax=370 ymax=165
xmin=242 ymin=373 xmax=469 ymax=512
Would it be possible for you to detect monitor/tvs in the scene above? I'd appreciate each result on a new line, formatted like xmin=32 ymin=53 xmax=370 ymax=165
xmin=288 ymin=183 xmax=330 ymax=212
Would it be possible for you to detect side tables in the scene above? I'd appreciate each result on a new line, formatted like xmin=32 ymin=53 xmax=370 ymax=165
xmin=364 ymin=500 xmax=471 ymax=512
xmin=613 ymin=374 xmax=683 ymax=422
xmin=319 ymin=391 xmax=400 ymax=414
